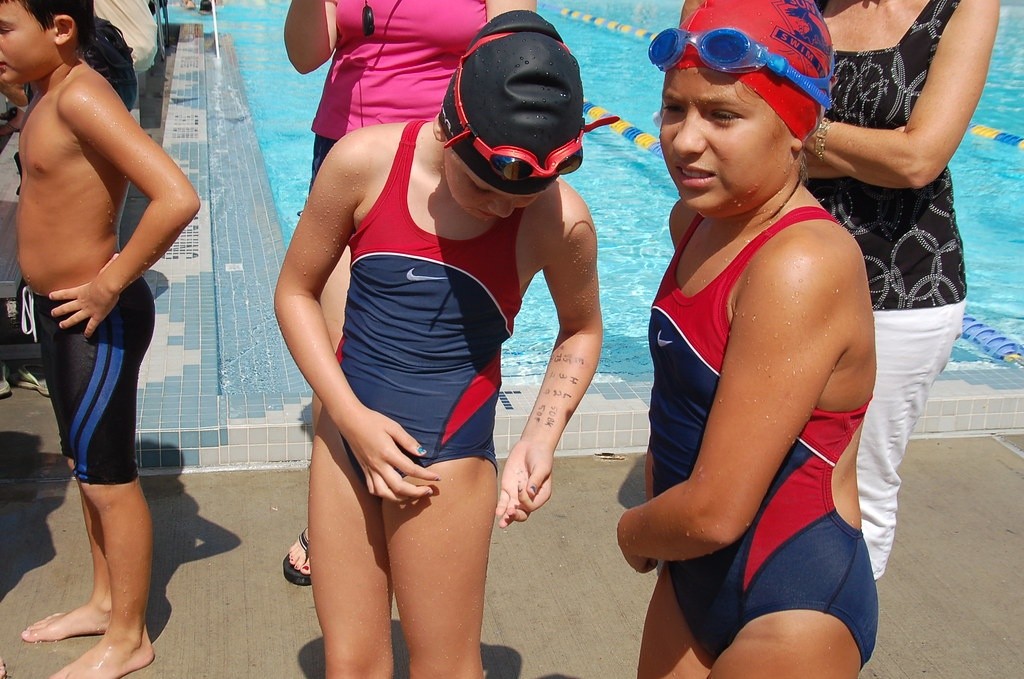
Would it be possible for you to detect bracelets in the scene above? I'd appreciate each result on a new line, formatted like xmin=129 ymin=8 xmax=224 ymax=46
xmin=815 ymin=118 xmax=830 ymax=161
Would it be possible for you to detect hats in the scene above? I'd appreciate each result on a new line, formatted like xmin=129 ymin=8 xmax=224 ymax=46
xmin=666 ymin=0 xmax=832 ymax=141
xmin=438 ymin=10 xmax=584 ymax=195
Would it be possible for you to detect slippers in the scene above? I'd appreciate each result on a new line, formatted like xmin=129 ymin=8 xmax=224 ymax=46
xmin=283 ymin=527 xmax=312 ymax=586
xmin=0 ymin=107 xmax=17 ymax=120
xmin=16 ymin=362 xmax=51 ymax=398
xmin=0 ymin=123 xmax=20 ymax=138
xmin=0 ymin=361 xmax=11 ymax=396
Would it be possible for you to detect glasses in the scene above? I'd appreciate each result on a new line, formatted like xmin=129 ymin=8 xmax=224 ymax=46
xmin=649 ymin=28 xmax=768 ymax=73
xmin=489 ymin=139 xmax=583 ymax=182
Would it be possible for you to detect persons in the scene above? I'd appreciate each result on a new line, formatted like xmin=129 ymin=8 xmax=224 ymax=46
xmin=792 ymin=0 xmax=999 ymax=600
xmin=0 ymin=0 xmax=200 ymax=679
xmin=282 ymin=0 xmax=554 ymax=587
xmin=276 ymin=11 xmax=604 ymax=679
xmin=616 ymin=0 xmax=880 ymax=679
